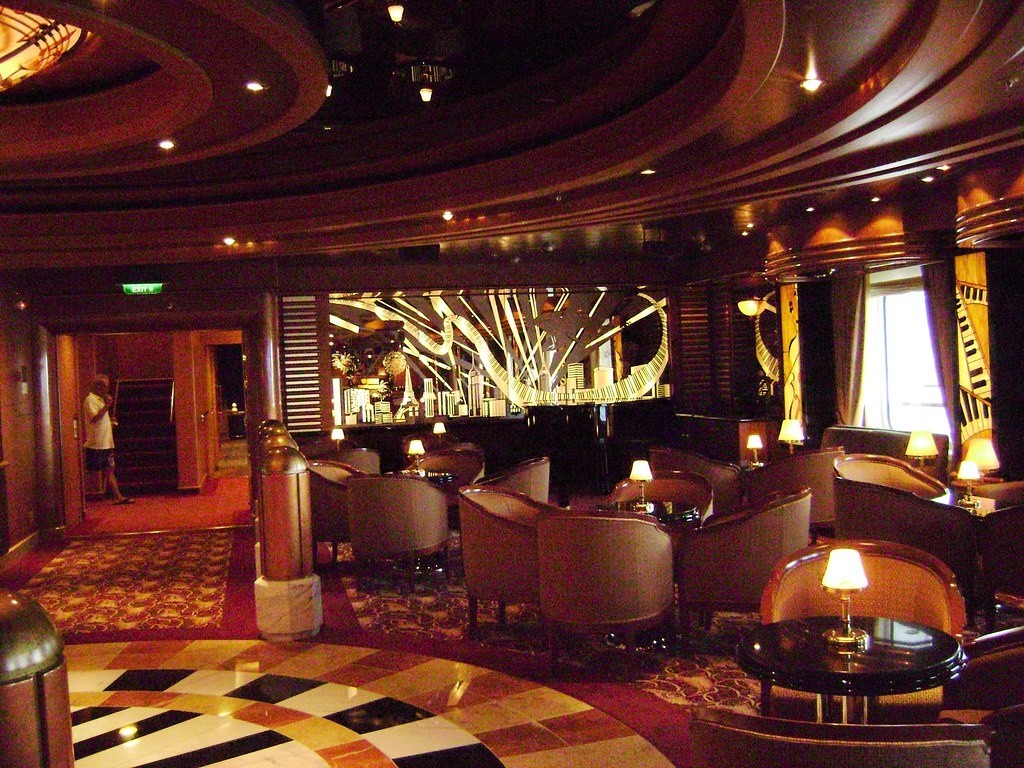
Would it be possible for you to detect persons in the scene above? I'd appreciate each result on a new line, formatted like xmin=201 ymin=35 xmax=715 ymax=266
xmin=83 ymin=374 xmax=136 ymax=505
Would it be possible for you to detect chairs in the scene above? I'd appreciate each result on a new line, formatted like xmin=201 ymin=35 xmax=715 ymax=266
xmin=297 ymin=442 xmax=1024 ymax=768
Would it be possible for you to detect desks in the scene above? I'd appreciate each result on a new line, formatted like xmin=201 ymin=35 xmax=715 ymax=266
xmin=595 ymin=499 xmax=700 ymax=652
xmin=729 ymin=460 xmax=770 ymax=473
xmin=926 ymin=493 xmax=1011 ymax=616
xmin=735 ymin=614 xmax=965 ymax=726
xmin=383 ymin=468 xmax=458 ymax=575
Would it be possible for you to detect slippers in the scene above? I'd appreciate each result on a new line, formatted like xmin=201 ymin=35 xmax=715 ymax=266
xmin=114 ymin=498 xmax=136 ymax=504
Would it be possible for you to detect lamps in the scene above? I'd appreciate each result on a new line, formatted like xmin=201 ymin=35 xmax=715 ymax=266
xmin=964 ymin=438 xmax=1000 ymax=486
xmin=407 ymin=439 xmax=426 ymax=479
xmin=0 ymin=6 xmax=89 ymax=92
xmin=433 ymin=421 xmax=447 ymax=450
xmin=958 ymin=461 xmax=981 ymax=510
xmin=778 ymin=419 xmax=806 ymax=455
xmin=820 ymin=548 xmax=872 ymax=656
xmin=904 ymin=430 xmax=939 ymax=474
xmin=747 ymin=434 xmax=763 ymax=469
xmin=630 ymin=459 xmax=655 ymax=513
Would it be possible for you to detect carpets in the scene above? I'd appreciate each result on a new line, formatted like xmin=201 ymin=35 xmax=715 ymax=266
xmin=0 ymin=524 xmax=261 ymax=643
